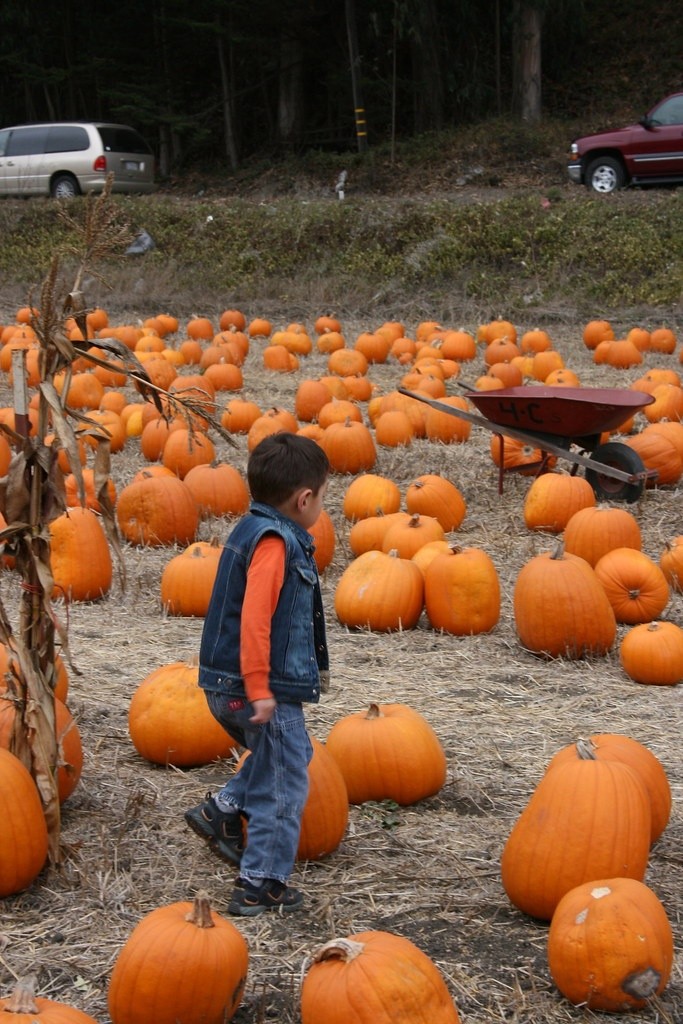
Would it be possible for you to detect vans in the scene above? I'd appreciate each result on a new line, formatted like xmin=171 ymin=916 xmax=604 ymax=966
xmin=0 ymin=123 xmax=160 ymax=198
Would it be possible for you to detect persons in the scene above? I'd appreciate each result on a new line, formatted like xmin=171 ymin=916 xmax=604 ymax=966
xmin=183 ymin=432 xmax=330 ymax=918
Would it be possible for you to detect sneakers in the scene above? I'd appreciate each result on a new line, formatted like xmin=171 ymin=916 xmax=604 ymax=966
xmin=186 ymin=792 xmax=244 ymax=869
xmin=229 ymin=877 xmax=305 ymax=915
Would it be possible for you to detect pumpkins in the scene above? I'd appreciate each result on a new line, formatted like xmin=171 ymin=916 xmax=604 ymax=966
xmin=0 ymin=307 xmax=683 ymax=1024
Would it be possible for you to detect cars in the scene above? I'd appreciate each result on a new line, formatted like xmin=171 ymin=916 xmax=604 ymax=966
xmin=566 ymin=92 xmax=683 ymax=193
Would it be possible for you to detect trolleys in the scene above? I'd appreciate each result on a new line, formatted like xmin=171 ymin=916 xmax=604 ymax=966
xmin=399 ymin=382 xmax=660 ymax=503
